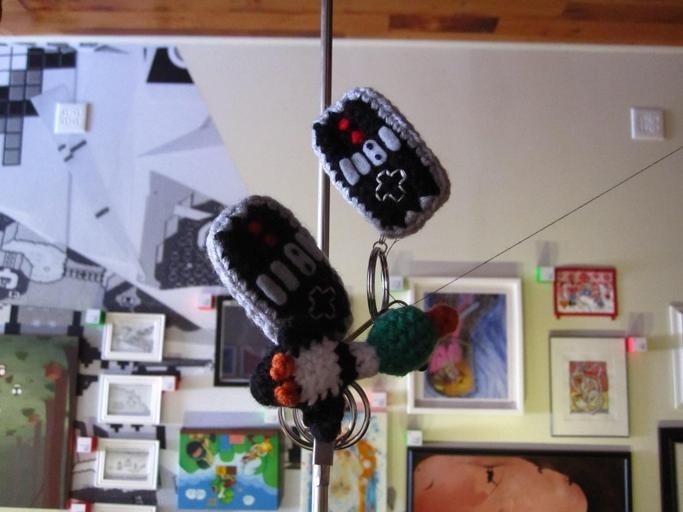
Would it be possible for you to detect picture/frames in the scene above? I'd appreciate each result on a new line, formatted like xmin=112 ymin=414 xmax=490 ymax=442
xmin=95 ymin=312 xmax=165 ymax=488
xmin=554 ymin=267 xmax=617 ymax=320
xmin=407 ymin=442 xmax=632 ymax=512
xmin=215 ymin=296 xmax=276 ymax=386
xmin=550 ymin=336 xmax=630 ymax=437
xmin=658 ymin=419 xmax=683 ymax=512
xmin=669 ymin=303 xmax=683 ymax=408
xmin=408 ymin=276 xmax=524 ymax=415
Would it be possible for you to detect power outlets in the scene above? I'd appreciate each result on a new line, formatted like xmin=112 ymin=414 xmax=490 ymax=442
xmin=54 ymin=103 xmax=87 ymax=133
xmin=631 ymin=108 xmax=665 ymax=141
